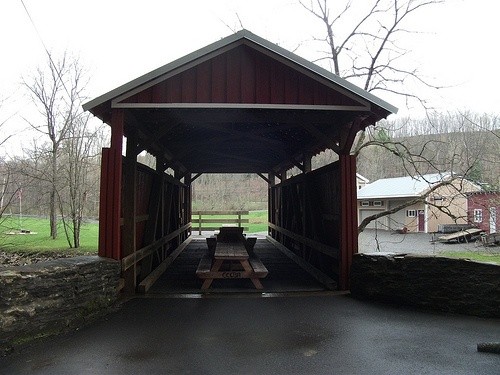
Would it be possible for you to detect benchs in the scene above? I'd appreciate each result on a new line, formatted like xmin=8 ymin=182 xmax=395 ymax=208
xmin=195 ymin=234 xmax=217 ymax=281
xmin=243 ymin=236 xmax=268 ymax=280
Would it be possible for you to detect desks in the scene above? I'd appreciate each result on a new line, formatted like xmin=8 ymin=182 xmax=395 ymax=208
xmin=198 ymin=225 xmax=265 ymax=293
